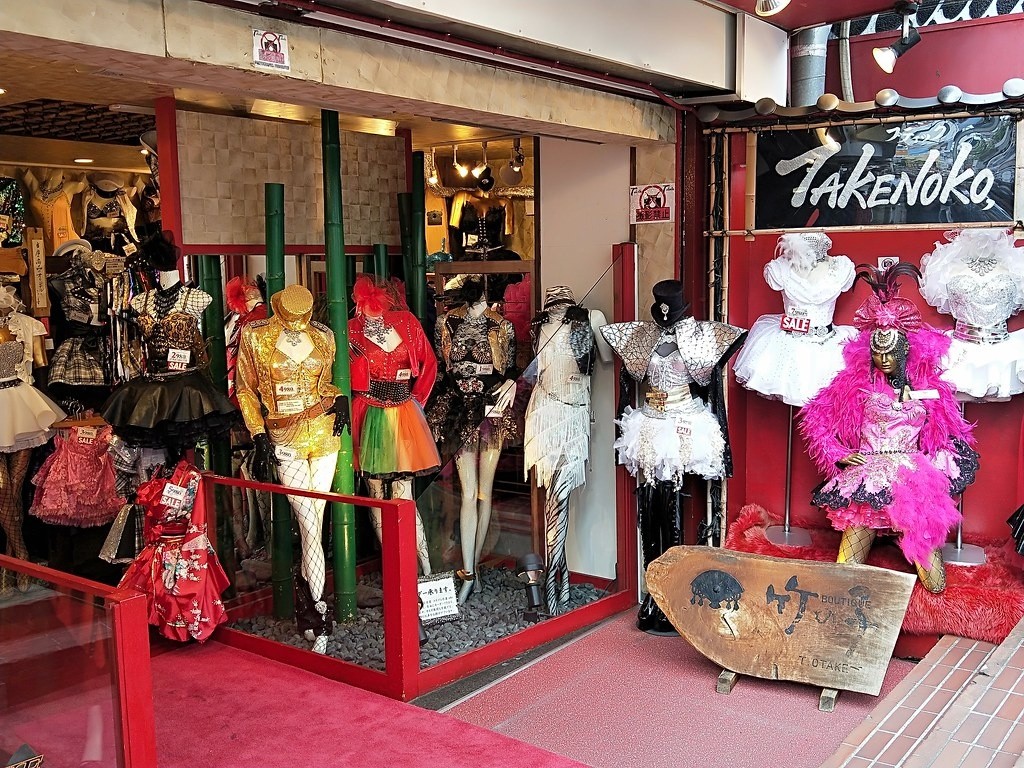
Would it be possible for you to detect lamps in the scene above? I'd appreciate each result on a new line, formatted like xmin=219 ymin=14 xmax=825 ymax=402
xmin=451 ymin=144 xmax=468 ymax=177
xmin=754 ymin=0 xmax=792 ymax=17
xmin=428 ymin=145 xmax=438 ymax=184
xmin=499 ymin=138 xmax=525 ymax=185
xmin=471 ymin=140 xmax=487 ymax=178
xmin=515 ymin=551 xmax=546 ymax=623
xmin=872 ymin=0 xmax=922 ymax=74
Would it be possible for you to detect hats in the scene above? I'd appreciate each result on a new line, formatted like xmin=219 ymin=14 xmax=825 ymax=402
xmin=53 ymin=238 xmax=93 ymax=257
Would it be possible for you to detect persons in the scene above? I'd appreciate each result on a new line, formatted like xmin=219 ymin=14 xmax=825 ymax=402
xmin=599 ymin=279 xmax=749 ymax=632
xmin=129 ymin=270 xmax=235 ymax=430
xmin=0 ymin=284 xmax=67 ymax=600
xmin=425 ymin=280 xmax=523 ymax=606
xmin=449 ymin=176 xmax=526 ymax=316
xmin=224 ymin=277 xmax=271 ymax=569
xmin=524 ymin=285 xmax=613 ymax=616
xmin=21 ymin=168 xmax=162 ymax=257
xmin=348 ymin=272 xmax=441 ymax=576
xmin=793 ymin=262 xmax=980 ymax=593
xmin=732 ymin=232 xmax=861 ymax=407
xmin=918 ymin=227 xmax=1024 ymax=403
xmin=235 ymin=285 xmax=351 ymax=654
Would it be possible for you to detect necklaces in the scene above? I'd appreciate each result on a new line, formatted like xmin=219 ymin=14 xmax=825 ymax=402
xmin=364 ymin=316 xmax=394 ymax=344
xmin=284 ymin=327 xmax=301 ymax=346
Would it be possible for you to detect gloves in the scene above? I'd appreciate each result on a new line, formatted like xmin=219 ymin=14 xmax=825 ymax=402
xmin=253 ymin=434 xmax=281 ymax=482
xmin=326 ymin=395 xmax=352 ymax=437
